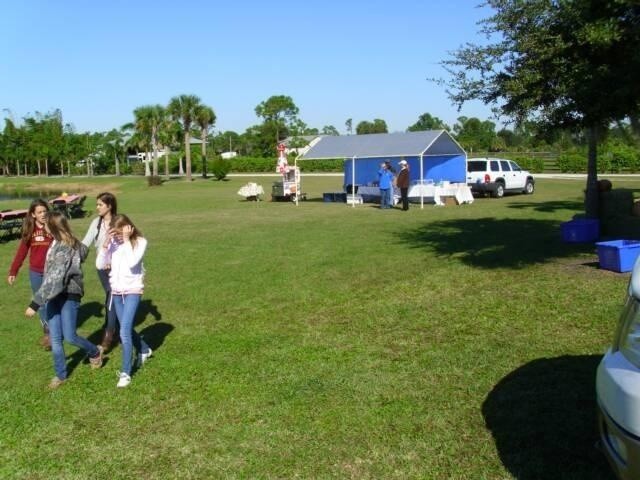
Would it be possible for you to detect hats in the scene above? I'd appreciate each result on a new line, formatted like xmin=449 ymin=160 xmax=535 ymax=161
xmin=397 ymin=160 xmax=408 ymax=165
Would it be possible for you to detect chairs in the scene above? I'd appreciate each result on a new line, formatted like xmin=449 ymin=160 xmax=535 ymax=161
xmin=0 ymin=193 xmax=88 ymax=243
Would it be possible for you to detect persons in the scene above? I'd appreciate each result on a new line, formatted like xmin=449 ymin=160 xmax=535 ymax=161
xmin=95 ymin=214 xmax=152 ymax=388
xmin=377 ymin=160 xmax=409 ymax=211
xmin=81 ymin=192 xmax=118 ymax=354
xmin=8 ymin=200 xmax=54 ymax=351
xmin=25 ymin=212 xmax=104 ymax=389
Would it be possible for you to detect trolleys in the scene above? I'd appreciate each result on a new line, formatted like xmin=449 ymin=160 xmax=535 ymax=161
xmin=271 ymin=166 xmax=301 ymax=202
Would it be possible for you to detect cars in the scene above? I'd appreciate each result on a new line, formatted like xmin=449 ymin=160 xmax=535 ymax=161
xmin=593 ymin=248 xmax=640 ymax=480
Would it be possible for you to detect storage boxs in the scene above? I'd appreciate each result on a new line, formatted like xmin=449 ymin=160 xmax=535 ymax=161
xmin=594 ymin=239 xmax=640 ymax=273
xmin=445 ymin=197 xmax=457 ymax=206
xmin=322 ymin=184 xmax=363 ymax=205
xmin=560 ymin=217 xmax=600 ymax=243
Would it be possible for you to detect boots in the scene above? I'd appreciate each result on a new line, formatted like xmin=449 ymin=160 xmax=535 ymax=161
xmin=101 ymin=329 xmax=116 ymax=353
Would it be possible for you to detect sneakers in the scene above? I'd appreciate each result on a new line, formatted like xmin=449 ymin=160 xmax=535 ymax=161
xmin=116 ymin=370 xmax=132 ymax=388
xmin=134 ymin=348 xmax=153 ymax=368
xmin=48 ymin=376 xmax=67 ymax=388
xmin=37 ymin=335 xmax=51 ymax=351
xmin=89 ymin=345 xmax=104 ymax=369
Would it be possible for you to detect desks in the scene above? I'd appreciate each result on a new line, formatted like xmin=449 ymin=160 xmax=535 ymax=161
xmin=356 ymin=184 xmax=470 ymax=207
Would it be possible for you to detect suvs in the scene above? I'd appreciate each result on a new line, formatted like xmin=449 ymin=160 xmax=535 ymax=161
xmin=465 ymin=156 xmax=538 ymax=199
